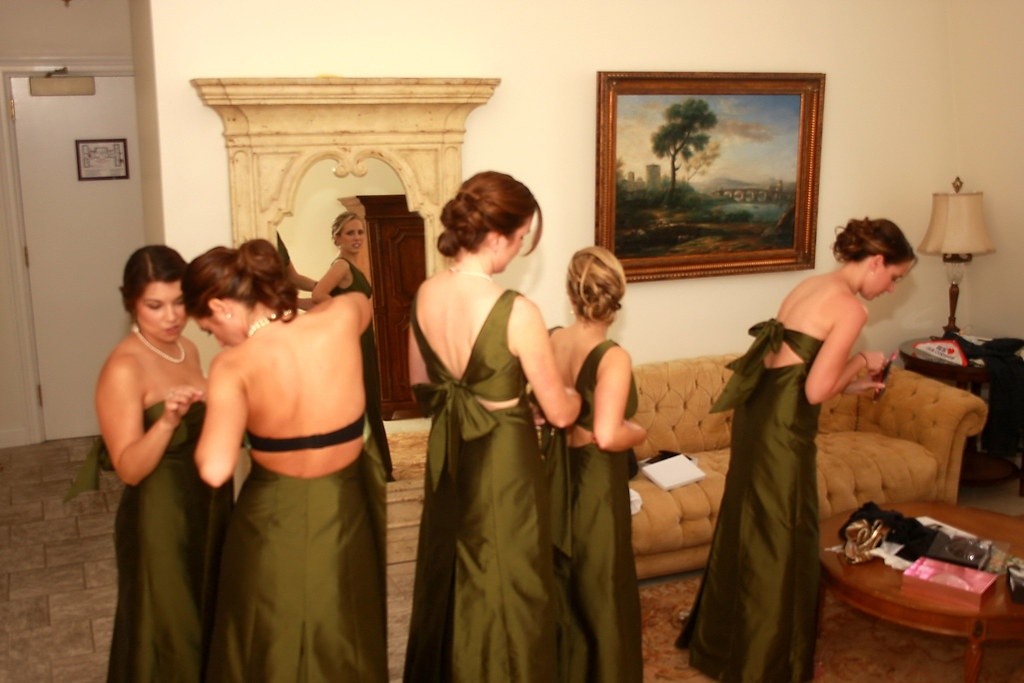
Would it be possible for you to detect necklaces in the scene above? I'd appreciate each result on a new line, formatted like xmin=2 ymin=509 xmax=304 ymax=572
xmin=246 ymin=306 xmax=307 ymax=338
xmin=447 ymin=266 xmax=494 ymax=282
xmin=130 ymin=321 xmax=186 ymax=364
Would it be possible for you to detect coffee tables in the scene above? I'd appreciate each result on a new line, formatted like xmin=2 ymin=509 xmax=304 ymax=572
xmin=819 ymin=501 xmax=1024 ymax=683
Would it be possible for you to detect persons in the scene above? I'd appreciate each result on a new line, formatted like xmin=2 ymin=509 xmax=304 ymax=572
xmin=403 ymin=170 xmax=582 ymax=683
xmin=674 ymin=218 xmax=918 ymax=683
xmin=311 ymin=210 xmax=395 ymax=483
xmin=180 ymin=240 xmax=391 ymax=683
xmin=95 ymin=243 xmax=234 ymax=683
xmin=531 ymin=246 xmax=648 ymax=683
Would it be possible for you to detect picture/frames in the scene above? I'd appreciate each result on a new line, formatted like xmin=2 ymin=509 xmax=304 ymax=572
xmin=595 ymin=71 xmax=825 ymax=283
xmin=76 ymin=139 xmax=129 ymax=181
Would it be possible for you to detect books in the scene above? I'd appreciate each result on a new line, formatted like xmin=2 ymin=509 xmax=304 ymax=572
xmin=642 ymin=453 xmax=705 ymax=492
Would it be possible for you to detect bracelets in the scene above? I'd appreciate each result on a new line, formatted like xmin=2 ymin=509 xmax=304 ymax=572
xmin=858 ymin=352 xmax=868 ymax=368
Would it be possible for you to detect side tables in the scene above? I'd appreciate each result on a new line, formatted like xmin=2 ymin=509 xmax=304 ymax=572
xmin=900 ymin=338 xmax=1019 ymax=486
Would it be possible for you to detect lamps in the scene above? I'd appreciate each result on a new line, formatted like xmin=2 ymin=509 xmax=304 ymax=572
xmin=917 ymin=177 xmax=995 ymax=342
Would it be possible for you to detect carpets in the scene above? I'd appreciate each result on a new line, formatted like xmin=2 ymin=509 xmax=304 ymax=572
xmin=640 ymin=569 xmax=1024 ymax=682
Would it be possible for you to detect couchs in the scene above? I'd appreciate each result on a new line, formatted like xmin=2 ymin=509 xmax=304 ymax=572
xmin=630 ymin=355 xmax=988 ymax=579
